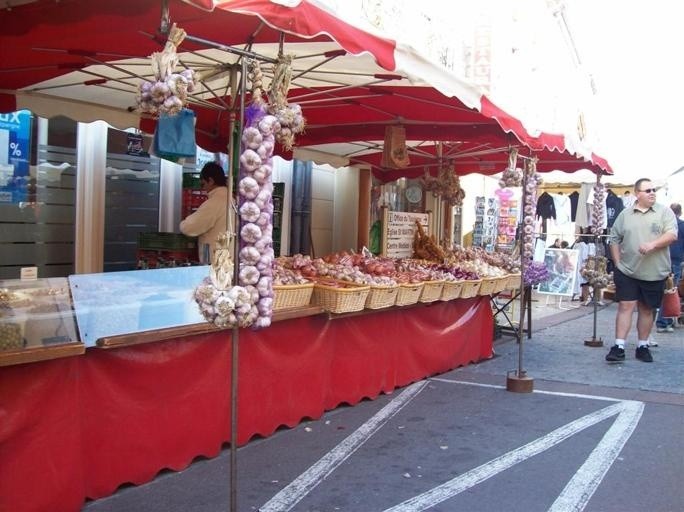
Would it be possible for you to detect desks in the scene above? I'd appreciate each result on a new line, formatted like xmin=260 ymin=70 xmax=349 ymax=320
xmin=0 ymin=294 xmax=498 ymax=511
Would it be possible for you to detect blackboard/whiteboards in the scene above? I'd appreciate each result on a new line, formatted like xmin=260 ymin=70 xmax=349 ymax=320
xmin=536 ymin=248 xmax=580 ymax=296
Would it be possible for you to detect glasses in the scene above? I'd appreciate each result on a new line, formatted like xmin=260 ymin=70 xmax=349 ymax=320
xmin=647 ymin=189 xmax=655 ymax=192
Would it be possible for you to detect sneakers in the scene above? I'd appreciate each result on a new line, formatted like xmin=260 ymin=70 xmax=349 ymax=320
xmin=606 ymin=345 xmax=625 ymax=361
xmin=636 ymin=341 xmax=657 ymax=362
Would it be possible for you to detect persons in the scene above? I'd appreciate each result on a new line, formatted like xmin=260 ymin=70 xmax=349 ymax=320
xmin=656 ymin=204 xmax=684 ymax=332
xmin=647 ymin=334 xmax=658 ymax=347
xmin=180 ymin=163 xmax=236 ymax=265
xmin=606 ymin=178 xmax=679 ymax=362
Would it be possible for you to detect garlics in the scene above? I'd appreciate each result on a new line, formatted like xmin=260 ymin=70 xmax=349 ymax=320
xmin=591 ymin=185 xmax=604 ymax=235
xmin=140 ymin=70 xmax=200 ymax=120
xmin=195 ymin=104 xmax=305 ymax=333
xmin=499 ymin=167 xmax=543 ymax=269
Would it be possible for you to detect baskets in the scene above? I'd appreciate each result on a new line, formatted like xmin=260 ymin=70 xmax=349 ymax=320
xmin=272 ymin=273 xmax=521 ymax=314
